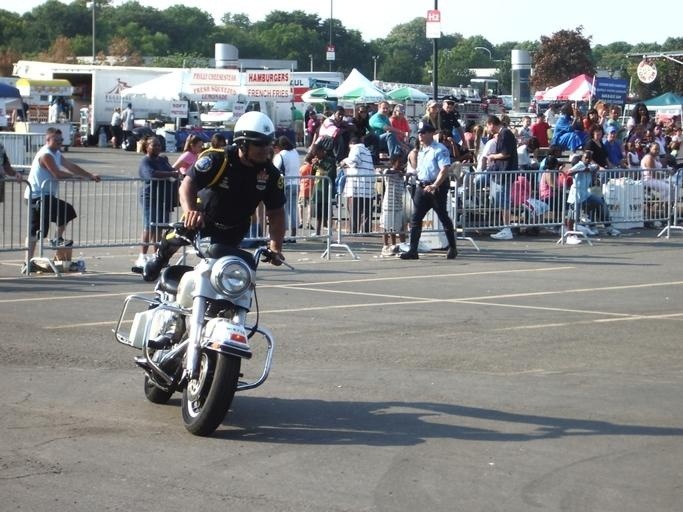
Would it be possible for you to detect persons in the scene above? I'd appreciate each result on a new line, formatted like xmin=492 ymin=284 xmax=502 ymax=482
xmin=141 ymin=109 xmax=288 ymax=285
xmin=24 ymin=127 xmax=104 ymax=274
xmin=108 ymin=98 xmax=682 ymax=272
xmin=0 ymin=143 xmax=23 ymax=204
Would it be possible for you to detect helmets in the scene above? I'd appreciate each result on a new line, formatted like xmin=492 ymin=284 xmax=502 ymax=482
xmin=233 ymin=111 xmax=276 ymax=143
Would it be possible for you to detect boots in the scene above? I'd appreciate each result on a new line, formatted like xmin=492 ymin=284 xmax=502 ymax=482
xmin=400 ymin=227 xmax=421 ymax=259
xmin=142 ymin=228 xmax=182 ymax=281
xmin=444 ymin=229 xmax=457 ymax=259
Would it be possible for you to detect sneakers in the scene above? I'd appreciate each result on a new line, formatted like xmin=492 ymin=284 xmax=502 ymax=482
xmin=306 ymin=232 xmax=323 ymax=241
xmin=381 ymin=247 xmax=395 ymax=256
xmin=566 ymin=235 xmax=582 ymax=245
xmin=604 ymin=225 xmax=621 ymax=236
xmin=462 ymin=199 xmax=479 ymax=208
xmin=22 ymin=260 xmax=49 ymax=272
xmin=49 ymin=236 xmax=73 ymax=250
xmin=283 ymin=235 xmax=296 ymax=243
xmin=490 ymin=229 xmax=513 ymax=240
xmin=136 ymin=258 xmax=147 ymax=269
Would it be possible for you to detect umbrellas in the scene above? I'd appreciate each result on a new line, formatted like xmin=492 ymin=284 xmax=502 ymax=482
xmin=300 ymin=85 xmax=339 ymax=114
xmin=535 ymin=73 xmax=597 ymax=110
xmin=387 ymin=84 xmax=430 ymax=118
xmin=323 ymin=66 xmax=393 ymax=119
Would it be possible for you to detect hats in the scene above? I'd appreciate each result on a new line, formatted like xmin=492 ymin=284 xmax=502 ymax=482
xmin=429 ymin=101 xmax=439 ymax=108
xmin=313 ymin=136 xmax=334 ymax=150
xmin=418 ymin=120 xmax=434 ymax=133
xmin=442 ymin=95 xmax=458 ymax=104
xmin=607 ymin=126 xmax=616 ymax=133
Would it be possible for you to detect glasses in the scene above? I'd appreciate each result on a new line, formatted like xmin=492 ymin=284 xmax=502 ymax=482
xmin=252 ymin=141 xmax=272 ymax=147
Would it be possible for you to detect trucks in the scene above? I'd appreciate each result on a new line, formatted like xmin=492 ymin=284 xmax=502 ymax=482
xmin=199 ymin=71 xmax=344 ymax=144
xmin=47 ymin=66 xmax=202 ymax=146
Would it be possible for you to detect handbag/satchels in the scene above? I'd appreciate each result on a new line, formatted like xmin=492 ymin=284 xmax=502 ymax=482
xmin=280 ymin=164 xmax=285 ymax=176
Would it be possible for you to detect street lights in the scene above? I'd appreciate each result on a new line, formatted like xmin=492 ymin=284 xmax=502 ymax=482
xmin=372 ymin=54 xmax=379 ymax=81
xmin=474 ymin=46 xmax=492 ymax=61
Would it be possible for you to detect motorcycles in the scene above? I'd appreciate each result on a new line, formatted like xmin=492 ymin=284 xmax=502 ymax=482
xmin=110 ymin=221 xmax=295 ymax=438
